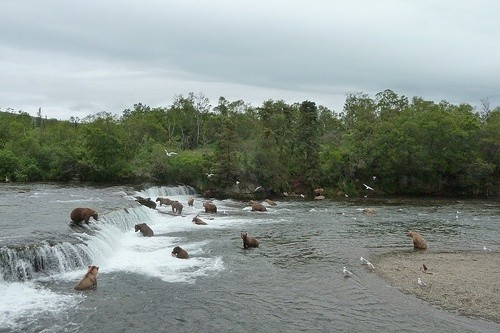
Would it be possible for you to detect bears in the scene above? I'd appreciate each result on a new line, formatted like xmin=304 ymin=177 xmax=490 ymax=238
xmin=202 ymin=201 xmax=218 ymax=215
xmin=70 ymin=207 xmax=99 ymax=227
xmin=241 ymin=232 xmax=259 ymax=250
xmin=156 ymin=197 xmax=183 ymax=215
xmin=249 ymin=198 xmax=277 ymax=213
xmin=404 ymin=231 xmax=429 ymax=251
xmin=134 ymin=223 xmax=154 ymax=237
xmin=191 ymin=215 xmax=209 ymax=226
xmin=187 ymin=197 xmax=195 ymax=206
xmin=312 ymin=188 xmax=324 ymax=197
xmin=74 ymin=265 xmax=100 ymax=291
xmin=172 ymin=245 xmax=189 ymax=259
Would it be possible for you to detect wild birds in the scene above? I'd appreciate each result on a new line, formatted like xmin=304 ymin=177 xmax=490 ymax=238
xmin=363 ymin=183 xmax=375 ymax=191
xmin=342 ymin=266 xmax=354 ymax=278
xmin=163 ymin=148 xmax=179 ymax=158
xmin=254 ymin=185 xmax=263 ymax=192
xmin=372 ymin=175 xmax=376 ymax=181
xmin=360 ymin=255 xmax=376 ymax=272
xmin=205 ymin=172 xmax=215 ymax=178
xmin=482 ymin=245 xmax=492 ymax=254
xmin=417 ymin=277 xmax=427 ymax=288
